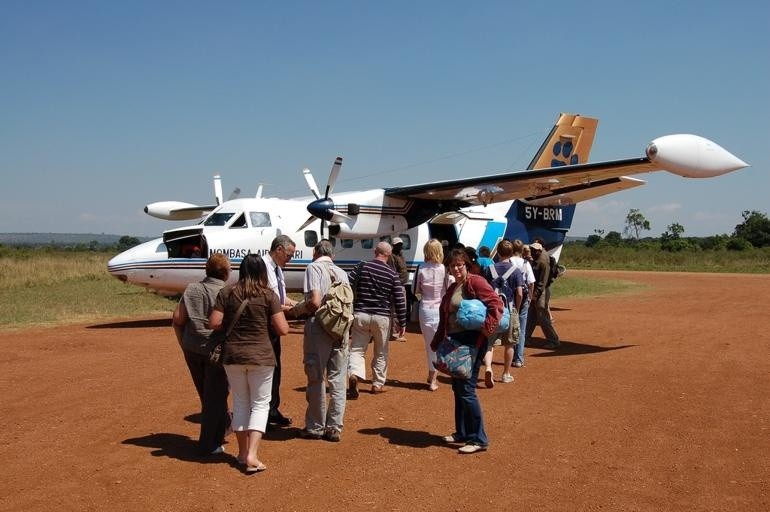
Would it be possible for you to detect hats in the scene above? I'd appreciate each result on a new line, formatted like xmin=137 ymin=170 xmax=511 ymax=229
xmin=530 ymin=243 xmax=543 ymax=251
xmin=392 ymin=237 xmax=403 ymax=244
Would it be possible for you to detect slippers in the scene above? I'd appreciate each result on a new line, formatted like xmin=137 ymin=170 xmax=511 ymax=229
xmin=246 ymin=461 xmax=267 ymax=472
xmin=235 ymin=454 xmax=247 ymax=464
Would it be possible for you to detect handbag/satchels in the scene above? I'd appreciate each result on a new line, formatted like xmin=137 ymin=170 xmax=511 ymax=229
xmin=209 ymin=344 xmax=223 ymax=368
xmin=314 ymin=281 xmax=354 ymax=344
xmin=432 ymin=336 xmax=479 ymax=380
xmin=546 ymin=256 xmax=558 ymax=288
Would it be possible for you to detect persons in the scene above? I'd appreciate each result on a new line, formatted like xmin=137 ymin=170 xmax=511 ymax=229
xmin=348 ymin=238 xmax=407 ymax=400
xmin=429 ymin=248 xmax=504 ymax=454
xmin=175 ymin=253 xmax=233 ymax=461
xmin=210 ymin=253 xmax=289 ymax=472
xmin=181 ymin=240 xmax=201 ymax=257
xmin=262 ymin=235 xmax=354 ymax=442
xmin=412 ymin=238 xmax=561 ymax=391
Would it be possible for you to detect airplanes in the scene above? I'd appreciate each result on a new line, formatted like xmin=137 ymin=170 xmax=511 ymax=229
xmin=107 ymin=112 xmax=752 ymax=325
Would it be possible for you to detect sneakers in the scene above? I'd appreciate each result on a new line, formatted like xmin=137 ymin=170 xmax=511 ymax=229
xmin=349 ymin=375 xmax=359 ymax=399
xmin=542 ymin=342 xmax=558 ymax=349
xmin=372 ymin=386 xmax=387 ymax=394
xmin=503 ymin=375 xmax=514 ymax=383
xmin=392 ymin=336 xmax=407 ymax=342
xmin=485 ymin=369 xmax=494 ymax=388
xmin=325 ymin=428 xmax=340 ymax=441
xmin=512 ymin=359 xmax=522 ymax=368
xmin=298 ymin=426 xmax=321 ymax=439
xmin=430 ymin=385 xmax=439 ymax=390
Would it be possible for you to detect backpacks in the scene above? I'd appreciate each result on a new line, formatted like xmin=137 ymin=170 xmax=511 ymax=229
xmin=486 ymin=264 xmax=515 ymax=312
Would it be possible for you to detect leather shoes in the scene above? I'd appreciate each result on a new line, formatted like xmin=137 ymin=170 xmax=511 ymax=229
xmin=268 ymin=410 xmax=291 ymax=424
